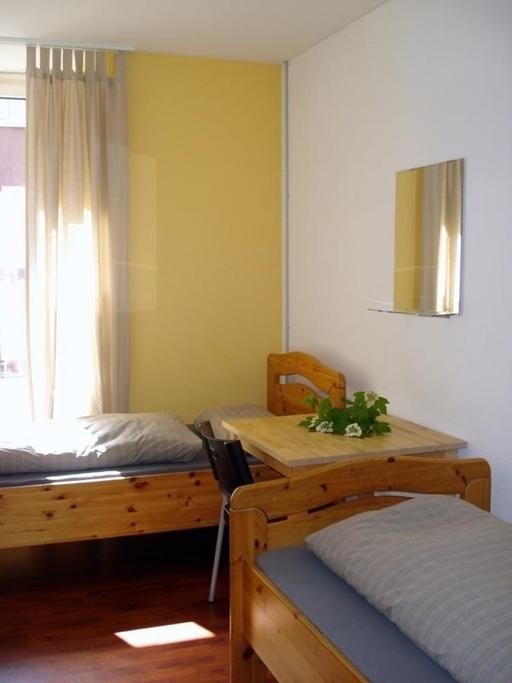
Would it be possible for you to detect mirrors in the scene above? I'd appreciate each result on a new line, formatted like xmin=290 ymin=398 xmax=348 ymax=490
xmin=392 ymin=158 xmax=463 ymax=317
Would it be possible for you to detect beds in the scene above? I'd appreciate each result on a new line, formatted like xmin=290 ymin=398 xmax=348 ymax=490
xmin=0 ymin=352 xmax=345 ymax=549
xmin=228 ymin=455 xmax=512 ymax=683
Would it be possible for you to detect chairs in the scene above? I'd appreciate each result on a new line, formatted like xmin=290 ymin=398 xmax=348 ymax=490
xmin=194 ymin=420 xmax=255 ymax=603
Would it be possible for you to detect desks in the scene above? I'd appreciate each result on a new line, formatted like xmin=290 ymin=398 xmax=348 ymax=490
xmin=221 ymin=405 xmax=468 ymax=477
